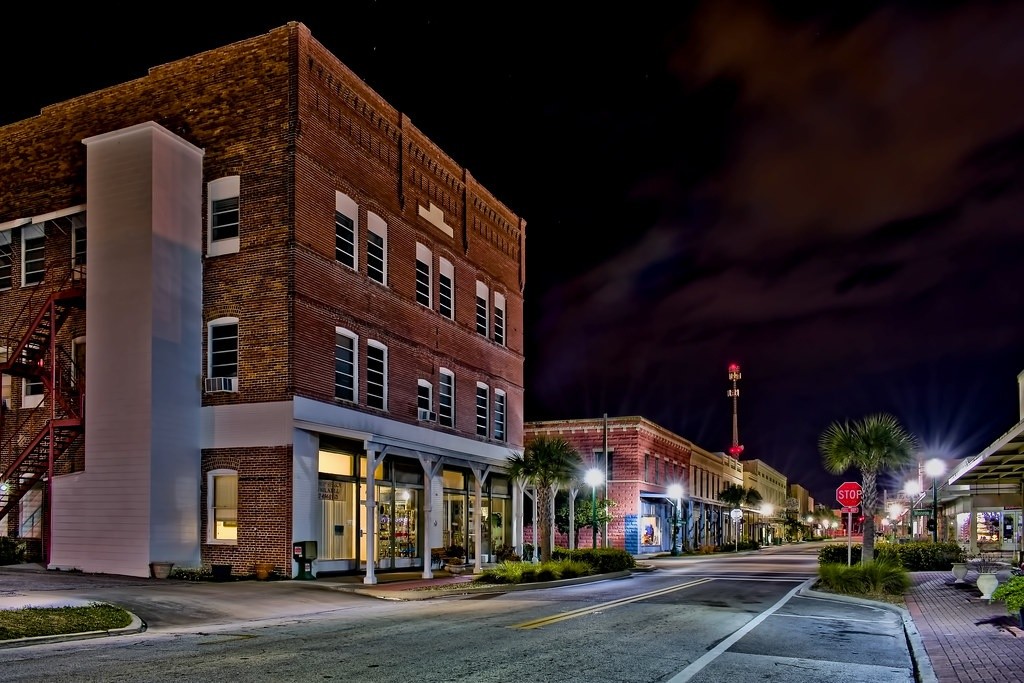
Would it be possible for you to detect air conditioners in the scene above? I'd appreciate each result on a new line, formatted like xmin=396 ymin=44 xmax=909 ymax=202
xmin=205 ymin=377 xmax=234 ymax=392
xmin=422 ymin=410 xmax=438 ymax=422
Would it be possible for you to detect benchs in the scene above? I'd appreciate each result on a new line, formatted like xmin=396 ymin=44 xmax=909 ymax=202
xmin=431 ymin=547 xmax=458 ymax=571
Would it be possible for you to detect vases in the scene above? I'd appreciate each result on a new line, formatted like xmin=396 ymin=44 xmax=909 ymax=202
xmin=151 ymin=561 xmax=175 ymax=580
xmin=210 ymin=563 xmax=234 ymax=582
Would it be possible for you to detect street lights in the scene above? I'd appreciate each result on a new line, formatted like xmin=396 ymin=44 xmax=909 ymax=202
xmin=905 ymin=480 xmax=919 ymax=543
xmin=823 ymin=519 xmax=829 ymax=539
xmin=889 ymin=504 xmax=901 ymax=539
xmin=807 ymin=517 xmax=813 ymax=538
xmin=761 ymin=503 xmax=774 ymax=547
xmin=668 ymin=484 xmax=684 ymax=556
xmin=925 ymin=458 xmax=944 ymax=543
xmin=833 ymin=522 xmax=838 ymax=538
xmin=584 ymin=468 xmax=604 ymax=550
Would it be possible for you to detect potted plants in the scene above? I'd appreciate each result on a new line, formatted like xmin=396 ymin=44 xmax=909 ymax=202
xmin=977 ymin=558 xmax=998 ymax=599
xmin=951 ymin=557 xmax=968 ymax=583
xmin=445 ymin=558 xmax=466 ymax=577
xmin=510 ymin=555 xmax=524 ymax=565
xmin=990 ymin=576 xmax=1024 ymax=631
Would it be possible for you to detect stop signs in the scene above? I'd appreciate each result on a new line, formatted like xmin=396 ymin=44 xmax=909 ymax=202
xmin=836 ymin=482 xmax=864 ymax=508
